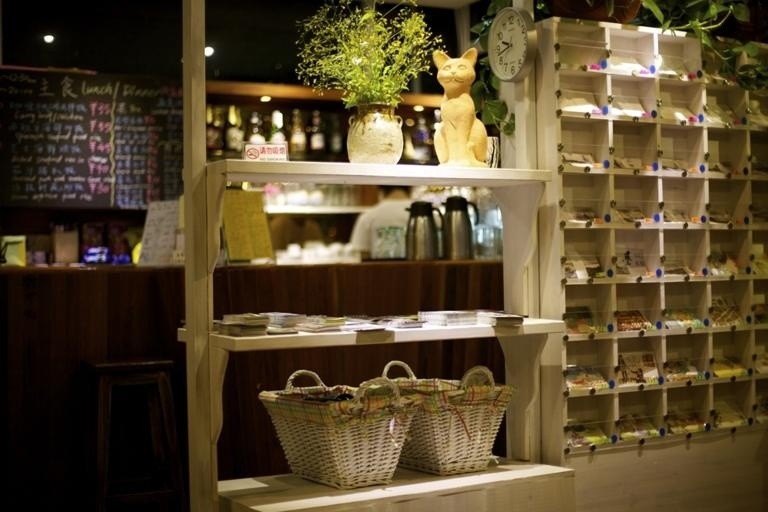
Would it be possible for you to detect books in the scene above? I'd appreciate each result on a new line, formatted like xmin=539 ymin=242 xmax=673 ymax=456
xmin=214 ymin=312 xmax=524 ymax=333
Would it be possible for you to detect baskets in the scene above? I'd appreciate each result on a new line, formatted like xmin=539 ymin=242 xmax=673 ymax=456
xmin=258 ymin=369 xmax=425 ymax=490
xmin=381 ymin=361 xmax=518 ymax=476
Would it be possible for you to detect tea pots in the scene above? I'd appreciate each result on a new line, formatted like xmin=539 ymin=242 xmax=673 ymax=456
xmin=404 ymin=200 xmax=442 ymax=261
xmin=438 ymin=196 xmax=478 ymax=259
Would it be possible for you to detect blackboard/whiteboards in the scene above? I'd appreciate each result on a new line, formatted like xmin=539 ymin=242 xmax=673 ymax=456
xmin=0 ymin=66 xmax=184 ymax=210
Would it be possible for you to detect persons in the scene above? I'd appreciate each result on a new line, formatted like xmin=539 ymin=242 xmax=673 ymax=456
xmin=350 ymin=181 xmax=434 ymax=266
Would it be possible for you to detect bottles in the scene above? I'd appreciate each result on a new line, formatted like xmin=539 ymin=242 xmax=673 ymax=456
xmin=204 ymin=104 xmax=440 ymax=164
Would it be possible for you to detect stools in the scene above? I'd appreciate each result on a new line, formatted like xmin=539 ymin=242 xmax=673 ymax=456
xmin=87 ymin=355 xmax=186 ymax=511
